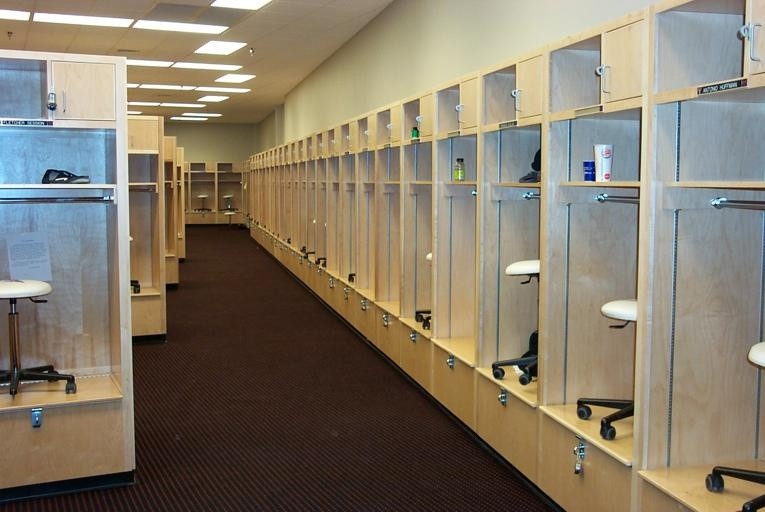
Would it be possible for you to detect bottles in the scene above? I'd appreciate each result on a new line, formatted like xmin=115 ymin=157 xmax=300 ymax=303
xmin=412 ymin=127 xmax=419 ymax=139
xmin=454 ymin=157 xmax=466 ymax=181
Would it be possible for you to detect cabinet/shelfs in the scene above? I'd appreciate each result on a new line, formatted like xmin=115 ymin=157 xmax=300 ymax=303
xmin=185 ymin=161 xmax=244 ymax=224
xmin=1 ymin=51 xmax=184 ymax=501
xmin=239 ymin=0 xmax=765 ymax=511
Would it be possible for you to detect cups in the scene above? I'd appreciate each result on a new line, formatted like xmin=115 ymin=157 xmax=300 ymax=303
xmin=582 ymin=145 xmax=613 ymax=181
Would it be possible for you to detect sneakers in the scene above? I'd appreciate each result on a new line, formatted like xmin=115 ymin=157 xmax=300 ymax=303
xmin=42 ymin=169 xmax=90 ymax=183
